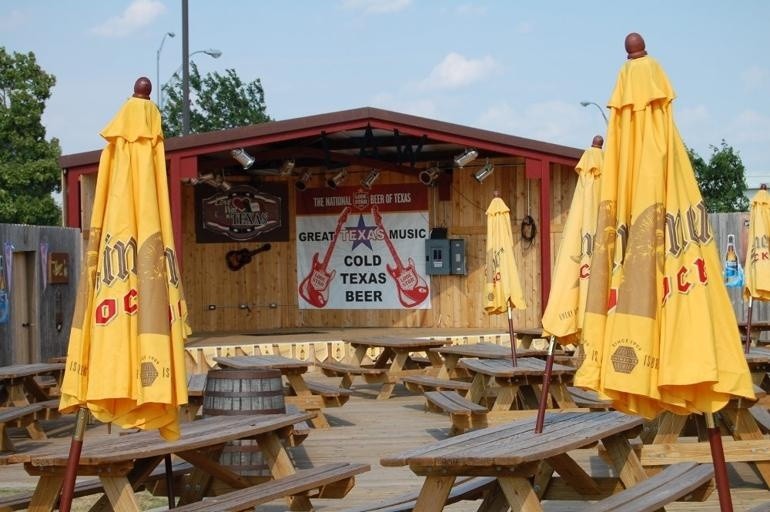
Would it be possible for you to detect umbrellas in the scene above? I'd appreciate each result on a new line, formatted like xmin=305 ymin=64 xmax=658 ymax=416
xmin=481 ymin=190 xmax=526 ymax=367
xmin=59 ymin=75 xmax=190 ymax=511
xmin=535 ymin=135 xmax=606 ymax=432
xmin=745 ymin=183 xmax=770 ymax=353
xmin=572 ymin=33 xmax=759 ymax=512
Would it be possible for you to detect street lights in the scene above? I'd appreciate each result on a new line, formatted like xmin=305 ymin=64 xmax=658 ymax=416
xmin=159 ymin=47 xmax=221 ymax=130
xmin=157 ymin=30 xmax=178 ymax=126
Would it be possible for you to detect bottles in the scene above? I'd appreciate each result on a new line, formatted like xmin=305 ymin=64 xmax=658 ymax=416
xmin=725 ymin=234 xmax=738 ymax=283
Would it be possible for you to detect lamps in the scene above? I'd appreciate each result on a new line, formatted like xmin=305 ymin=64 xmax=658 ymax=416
xmin=293 ymin=163 xmax=443 ymax=192
xmin=229 ymin=143 xmax=296 ymax=179
xmin=453 ymin=145 xmax=498 ymax=185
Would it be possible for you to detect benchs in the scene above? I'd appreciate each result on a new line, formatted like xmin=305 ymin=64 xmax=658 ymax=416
xmin=283 ymin=382 xmax=354 ymax=406
xmin=583 ymin=462 xmax=715 ymax=511
xmin=2 ymin=459 xmax=197 ymax=511
xmin=355 ymin=477 xmax=498 ymax=511
xmin=169 ymin=461 xmax=371 ymax=511
xmin=1 ymin=398 xmax=61 ymax=452
xmin=316 ymin=363 xmax=390 ymax=384
xmin=566 ymin=386 xmax=613 ymax=412
xmin=399 ymin=376 xmax=494 ymax=437
xmin=285 ymin=404 xmax=310 ymax=446
xmin=185 ymin=371 xmax=208 ymax=422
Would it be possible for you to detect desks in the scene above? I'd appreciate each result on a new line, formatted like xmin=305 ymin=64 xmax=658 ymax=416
xmin=2 ymin=357 xmax=67 ymax=441
xmin=2 ymin=411 xmax=318 ymax=512
xmin=514 ymin=328 xmax=562 ymax=350
xmin=343 ymin=335 xmax=453 ymax=400
xmin=641 ymin=320 xmax=770 ymax=502
xmin=429 ymin=343 xmax=578 ymax=412
xmin=212 ymin=354 xmax=330 ymax=429
xmin=379 ymin=410 xmax=665 ymax=512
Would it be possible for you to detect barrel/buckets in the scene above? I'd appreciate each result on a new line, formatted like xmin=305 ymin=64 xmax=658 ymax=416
xmin=202 ymin=370 xmax=285 ymax=477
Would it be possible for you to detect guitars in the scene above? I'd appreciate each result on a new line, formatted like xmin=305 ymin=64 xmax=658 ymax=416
xmin=372 ymin=205 xmax=428 ymax=308
xmin=299 ymin=204 xmax=351 ymax=308
xmin=226 ymin=243 xmax=272 ymax=271
